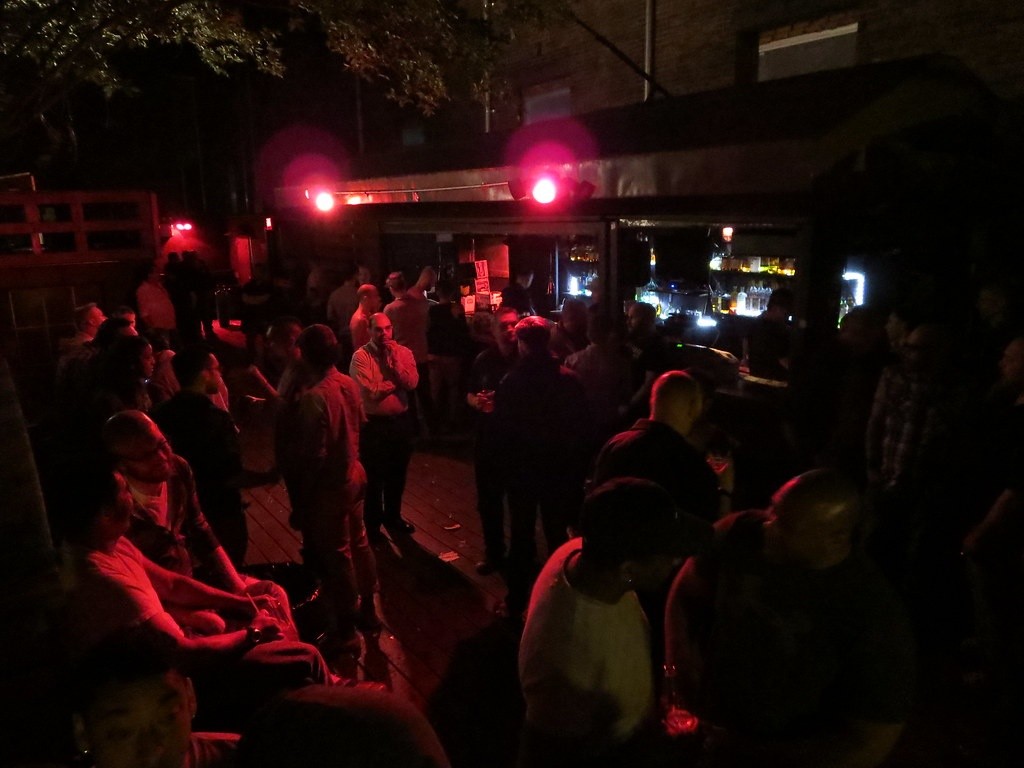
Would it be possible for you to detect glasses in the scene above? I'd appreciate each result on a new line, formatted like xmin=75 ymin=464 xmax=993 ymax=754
xmin=121 ymin=436 xmax=174 ymax=462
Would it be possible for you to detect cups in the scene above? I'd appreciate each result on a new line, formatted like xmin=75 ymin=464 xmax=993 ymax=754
xmin=713 ymin=251 xmax=796 ymax=276
xmin=479 ymin=390 xmax=495 ymax=412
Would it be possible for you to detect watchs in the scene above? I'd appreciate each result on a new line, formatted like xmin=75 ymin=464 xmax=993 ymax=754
xmin=246 ymin=624 xmax=263 ymax=643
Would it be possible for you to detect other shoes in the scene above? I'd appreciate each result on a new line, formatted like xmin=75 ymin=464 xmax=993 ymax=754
xmin=384 ymin=516 xmax=414 ymax=533
xmin=367 ymin=529 xmax=389 ymax=545
xmin=477 ymin=555 xmax=504 ymax=576
xmin=356 ymin=617 xmax=387 ymax=633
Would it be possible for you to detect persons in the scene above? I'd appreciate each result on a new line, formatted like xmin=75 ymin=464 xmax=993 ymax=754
xmin=58 ymin=250 xmax=471 ymax=768
xmin=468 ymin=287 xmax=1024 ymax=686
xmin=518 ymin=476 xmax=677 ymax=768
xmin=664 ymin=468 xmax=909 ymax=768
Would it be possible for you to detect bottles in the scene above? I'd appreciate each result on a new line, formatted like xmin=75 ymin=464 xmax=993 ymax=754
xmin=712 ymin=282 xmax=772 ymax=317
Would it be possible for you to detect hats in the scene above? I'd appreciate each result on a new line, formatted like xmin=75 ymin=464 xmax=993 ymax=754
xmin=585 ymin=477 xmax=713 ymax=562
